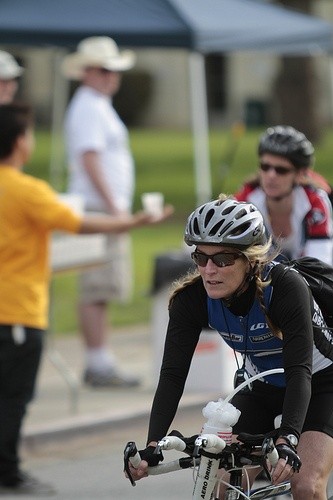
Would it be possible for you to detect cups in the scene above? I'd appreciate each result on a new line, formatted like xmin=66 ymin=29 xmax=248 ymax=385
xmin=199 ymin=425 xmax=232 ymax=447
xmin=142 ymin=193 xmax=162 ymax=219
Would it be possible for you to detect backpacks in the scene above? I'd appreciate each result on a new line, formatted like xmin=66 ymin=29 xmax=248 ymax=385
xmin=275 ymin=257 xmax=333 ymax=329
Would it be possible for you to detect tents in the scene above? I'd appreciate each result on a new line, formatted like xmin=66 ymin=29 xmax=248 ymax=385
xmin=0 ymin=0 xmax=333 ymax=210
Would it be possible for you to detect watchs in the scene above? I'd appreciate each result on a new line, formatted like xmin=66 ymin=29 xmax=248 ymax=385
xmin=279 ymin=434 xmax=299 ymax=448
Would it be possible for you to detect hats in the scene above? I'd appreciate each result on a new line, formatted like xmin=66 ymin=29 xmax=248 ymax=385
xmin=62 ymin=36 xmax=136 ymax=80
xmin=0 ymin=50 xmax=25 ymax=79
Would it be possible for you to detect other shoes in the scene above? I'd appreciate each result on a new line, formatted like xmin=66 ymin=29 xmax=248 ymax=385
xmin=0 ymin=475 xmax=56 ymax=495
xmin=82 ymin=368 xmax=141 ymax=388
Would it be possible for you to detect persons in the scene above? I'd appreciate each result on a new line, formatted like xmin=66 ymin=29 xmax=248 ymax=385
xmin=60 ymin=37 xmax=141 ymax=389
xmin=0 ymin=50 xmax=26 ymax=105
xmin=233 ymin=125 xmax=333 ymax=267
xmin=0 ymin=103 xmax=174 ymax=496
xmin=124 ymin=198 xmax=333 ymax=500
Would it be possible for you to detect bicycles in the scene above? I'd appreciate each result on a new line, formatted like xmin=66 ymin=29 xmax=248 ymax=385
xmin=122 ymin=434 xmax=292 ymax=500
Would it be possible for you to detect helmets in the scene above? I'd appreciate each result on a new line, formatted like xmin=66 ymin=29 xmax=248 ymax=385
xmin=259 ymin=126 xmax=315 ymax=165
xmin=184 ymin=199 xmax=265 ymax=246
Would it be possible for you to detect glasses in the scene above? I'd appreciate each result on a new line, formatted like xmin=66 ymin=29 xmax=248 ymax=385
xmin=191 ymin=252 xmax=243 ymax=268
xmin=260 ymin=163 xmax=293 ymax=175
